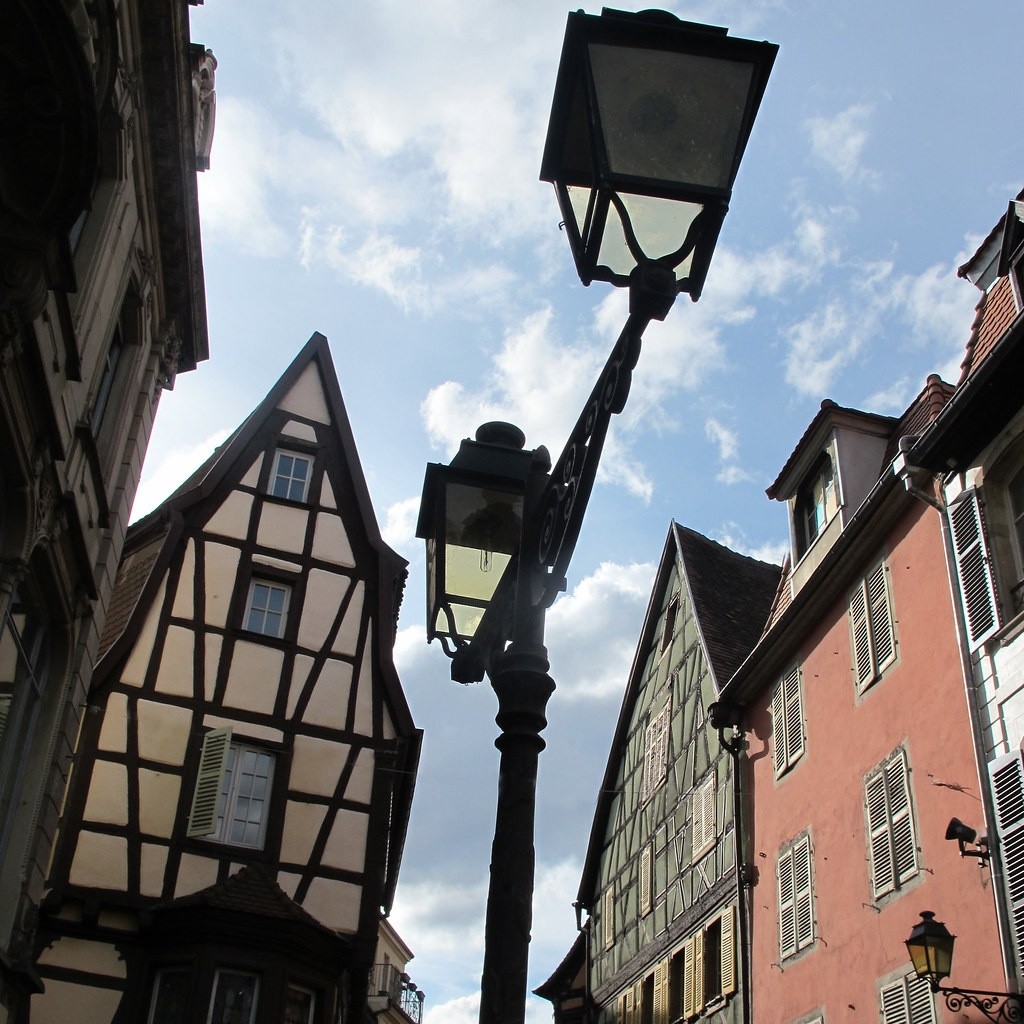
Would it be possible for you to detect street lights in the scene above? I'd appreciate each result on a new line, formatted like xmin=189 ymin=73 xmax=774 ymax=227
xmin=413 ymin=3 xmax=783 ymax=1023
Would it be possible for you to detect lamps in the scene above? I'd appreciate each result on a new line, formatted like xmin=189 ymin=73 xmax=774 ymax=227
xmin=942 ymin=817 xmax=990 ymax=866
xmin=902 ymin=909 xmax=1023 ymax=1024
